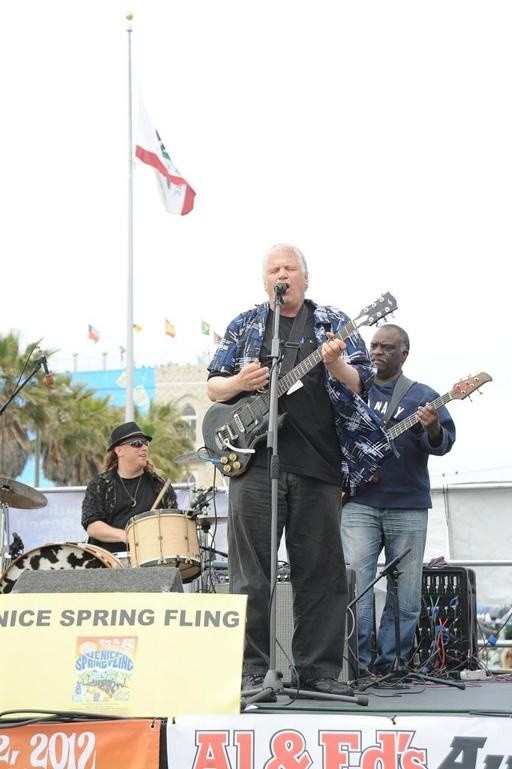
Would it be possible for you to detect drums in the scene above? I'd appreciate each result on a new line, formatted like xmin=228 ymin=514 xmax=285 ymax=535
xmin=124 ymin=508 xmax=204 ymax=583
xmin=0 ymin=541 xmax=123 ymax=594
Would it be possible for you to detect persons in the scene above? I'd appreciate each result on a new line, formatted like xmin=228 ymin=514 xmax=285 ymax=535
xmin=207 ymin=245 xmax=377 ymax=702
xmin=82 ymin=422 xmax=179 ymax=553
xmin=340 ymin=324 xmax=456 ymax=683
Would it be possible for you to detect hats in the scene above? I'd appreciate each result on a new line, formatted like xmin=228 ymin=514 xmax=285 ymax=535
xmin=105 ymin=421 xmax=152 ymax=453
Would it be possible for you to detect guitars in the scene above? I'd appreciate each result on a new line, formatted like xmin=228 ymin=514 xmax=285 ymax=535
xmin=388 ymin=372 xmax=494 ymax=455
xmin=201 ymin=289 xmax=400 ymax=479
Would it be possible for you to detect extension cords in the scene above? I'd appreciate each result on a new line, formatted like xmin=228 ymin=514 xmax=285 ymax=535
xmin=460 ymin=669 xmax=492 ymax=681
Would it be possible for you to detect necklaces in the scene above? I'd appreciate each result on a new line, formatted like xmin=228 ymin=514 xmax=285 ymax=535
xmin=116 ymin=470 xmax=144 ymax=507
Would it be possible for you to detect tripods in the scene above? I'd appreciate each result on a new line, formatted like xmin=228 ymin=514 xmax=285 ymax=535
xmin=346 ymin=545 xmax=465 ymax=690
xmin=239 ymin=290 xmax=370 ymax=709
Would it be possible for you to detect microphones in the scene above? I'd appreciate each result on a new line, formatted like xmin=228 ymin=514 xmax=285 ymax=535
xmin=274 ymin=282 xmax=288 ymax=294
xmin=190 ymin=486 xmax=212 ymax=507
xmin=37 ymin=348 xmax=53 ymax=386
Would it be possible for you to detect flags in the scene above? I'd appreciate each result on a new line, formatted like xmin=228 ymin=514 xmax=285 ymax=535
xmin=200 ymin=320 xmax=211 ymax=336
xmin=89 ymin=323 xmax=99 ymax=343
xmin=165 ymin=319 xmax=176 ymax=337
xmin=214 ymin=332 xmax=222 ymax=345
xmin=133 ymin=324 xmax=142 ymax=333
xmin=136 ymin=128 xmax=195 ymax=214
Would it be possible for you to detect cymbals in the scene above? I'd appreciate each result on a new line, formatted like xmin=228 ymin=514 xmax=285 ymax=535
xmin=0 ymin=476 xmax=48 ymax=510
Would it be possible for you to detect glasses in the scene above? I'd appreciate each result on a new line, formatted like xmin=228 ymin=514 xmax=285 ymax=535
xmin=121 ymin=440 xmax=151 ymax=448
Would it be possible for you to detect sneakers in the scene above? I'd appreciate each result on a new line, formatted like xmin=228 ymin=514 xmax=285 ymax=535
xmin=288 ymin=664 xmax=355 ymax=697
xmin=241 ymin=674 xmax=278 ymax=703
